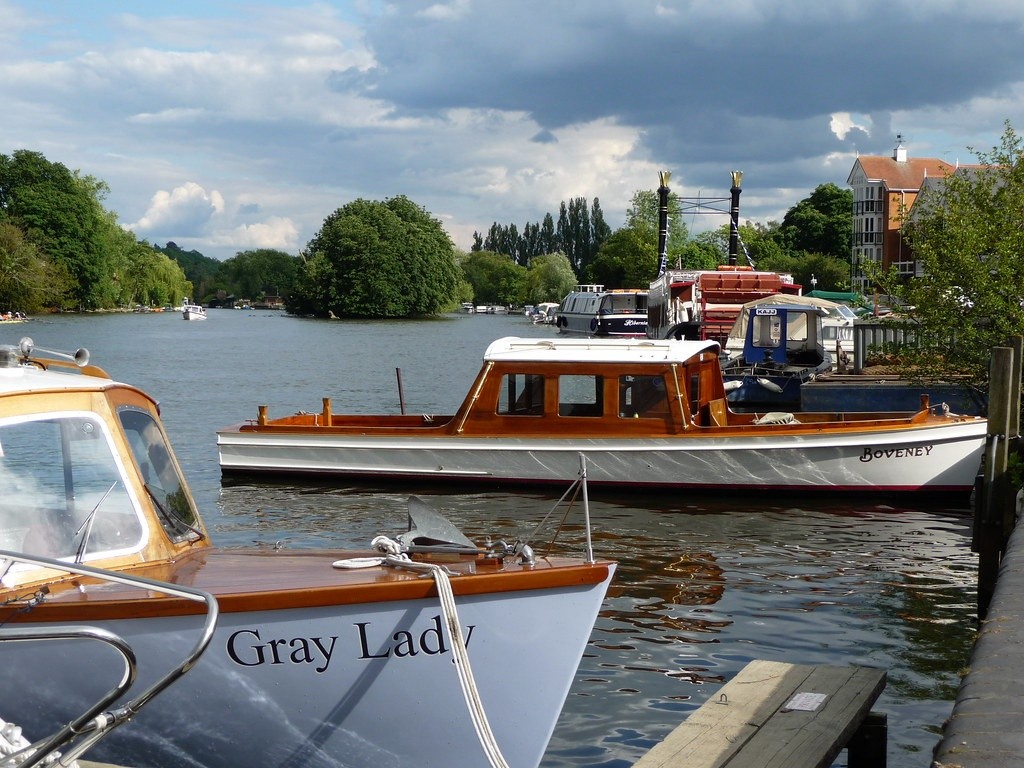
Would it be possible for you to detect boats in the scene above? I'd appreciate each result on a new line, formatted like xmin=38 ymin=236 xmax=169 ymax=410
xmin=645 ymin=169 xmax=989 ymax=420
xmin=554 ymin=285 xmax=650 ymax=336
xmin=0 ymin=338 xmax=621 ymax=768
xmin=183 ymin=304 xmax=206 ymax=321
xmin=523 ymin=302 xmax=561 ymax=325
xmin=0 ymin=315 xmax=29 ymax=325
xmin=458 ymin=302 xmax=509 ymax=315
xmin=218 ymin=335 xmax=986 ymax=509
